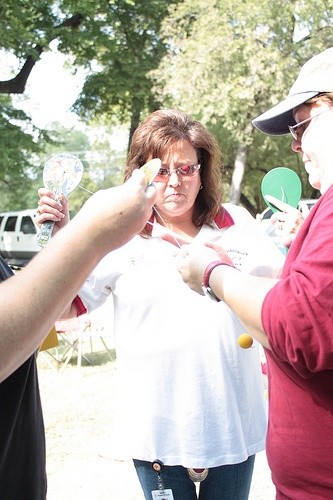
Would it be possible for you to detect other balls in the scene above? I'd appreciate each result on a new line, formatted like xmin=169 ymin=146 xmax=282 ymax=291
xmin=238 ymin=333 xmax=254 ymax=350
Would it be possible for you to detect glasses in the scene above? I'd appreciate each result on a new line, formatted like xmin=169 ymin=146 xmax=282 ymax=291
xmin=287 ymin=108 xmax=333 ymax=140
xmin=152 ymin=164 xmax=200 ymax=182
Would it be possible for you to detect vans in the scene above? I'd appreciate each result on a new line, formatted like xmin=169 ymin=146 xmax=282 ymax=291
xmin=0 ymin=209 xmax=43 ymax=265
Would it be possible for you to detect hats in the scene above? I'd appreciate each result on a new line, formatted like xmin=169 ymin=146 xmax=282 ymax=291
xmin=251 ymin=46 xmax=333 ymax=136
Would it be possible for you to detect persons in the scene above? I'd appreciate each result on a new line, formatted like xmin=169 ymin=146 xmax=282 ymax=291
xmin=36 ymin=107 xmax=279 ymax=500
xmin=182 ymin=47 xmax=333 ymax=500
xmin=0 ymin=156 xmax=159 ymax=500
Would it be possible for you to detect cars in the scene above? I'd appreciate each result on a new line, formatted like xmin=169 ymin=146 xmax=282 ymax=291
xmin=254 ymin=198 xmax=319 ymax=224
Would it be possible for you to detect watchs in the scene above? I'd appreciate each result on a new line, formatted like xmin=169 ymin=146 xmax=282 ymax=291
xmin=201 ymin=261 xmax=237 ymax=303
